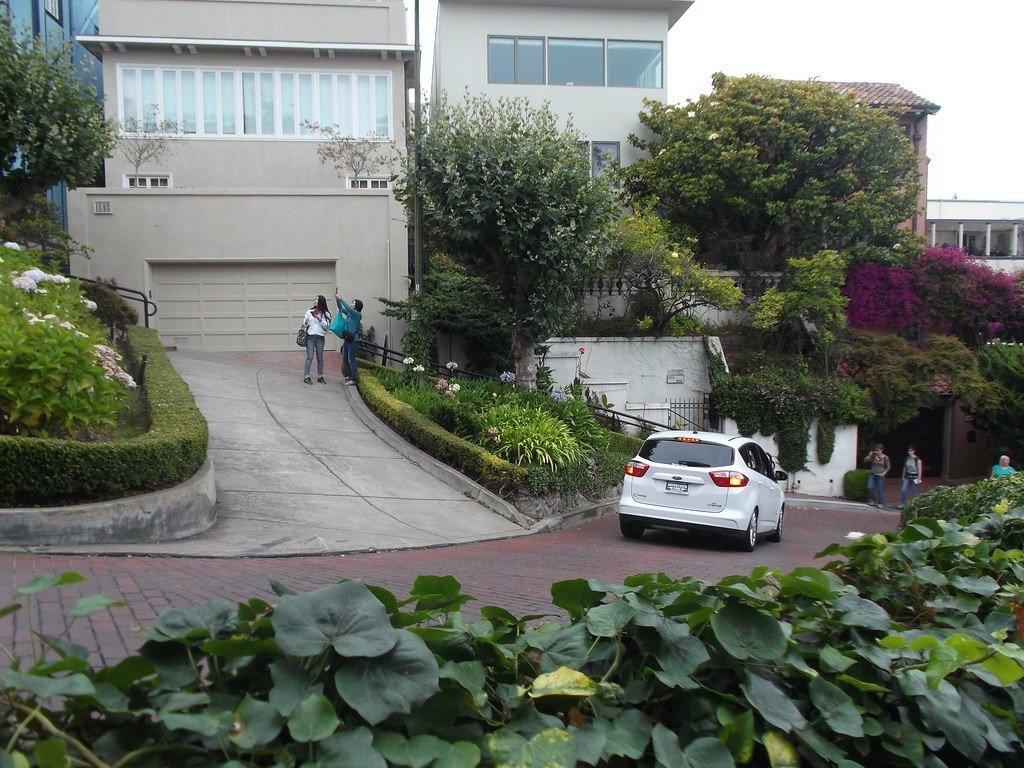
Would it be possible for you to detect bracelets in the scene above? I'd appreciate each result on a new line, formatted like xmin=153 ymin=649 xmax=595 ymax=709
xmin=919 ymin=479 xmax=921 ymax=480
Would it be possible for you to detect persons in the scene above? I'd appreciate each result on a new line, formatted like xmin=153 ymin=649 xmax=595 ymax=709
xmin=989 ymin=455 xmax=1016 ymax=479
xmin=303 ymin=295 xmax=332 ymax=384
xmin=335 ymin=293 xmax=364 ymax=386
xmin=895 ymin=446 xmax=922 ymax=509
xmin=864 ymin=444 xmax=890 ymax=509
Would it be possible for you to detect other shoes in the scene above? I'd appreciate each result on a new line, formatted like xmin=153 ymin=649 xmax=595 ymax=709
xmin=897 ymin=504 xmax=908 ymax=510
xmin=304 ymin=377 xmax=313 ymax=385
xmin=345 ymin=380 xmax=356 ymax=386
xmin=869 ymin=501 xmax=875 ymax=505
xmin=318 ymin=377 xmax=326 ymax=384
xmin=877 ymin=504 xmax=883 ymax=509
xmin=343 ymin=376 xmax=352 ymax=380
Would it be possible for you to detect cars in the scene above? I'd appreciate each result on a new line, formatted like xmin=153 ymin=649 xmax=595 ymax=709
xmin=616 ymin=431 xmax=788 ymax=552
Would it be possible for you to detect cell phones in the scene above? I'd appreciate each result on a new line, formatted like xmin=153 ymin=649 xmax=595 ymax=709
xmin=336 ymin=287 xmax=338 ymax=294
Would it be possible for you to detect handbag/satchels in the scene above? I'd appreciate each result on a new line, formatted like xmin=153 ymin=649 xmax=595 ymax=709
xmin=343 ymin=330 xmax=355 ymax=342
xmin=296 ymin=325 xmax=309 ymax=347
xmin=329 ymin=306 xmax=346 ymax=339
